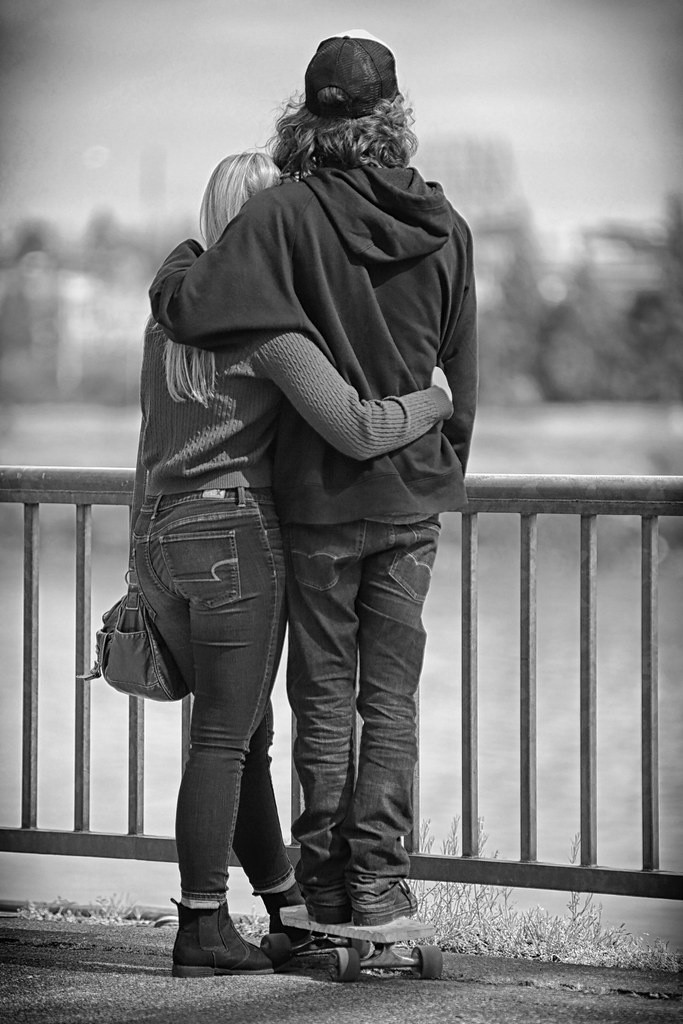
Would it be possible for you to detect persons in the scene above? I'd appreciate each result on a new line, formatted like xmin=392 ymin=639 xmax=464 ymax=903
xmin=147 ymin=34 xmax=476 ymax=930
xmin=128 ymin=150 xmax=452 ymax=977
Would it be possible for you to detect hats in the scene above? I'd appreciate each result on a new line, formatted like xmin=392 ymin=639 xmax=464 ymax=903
xmin=306 ymin=36 xmax=398 ymax=119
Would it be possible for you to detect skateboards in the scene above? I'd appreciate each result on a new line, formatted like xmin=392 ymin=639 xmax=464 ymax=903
xmin=258 ymin=902 xmax=443 ymax=983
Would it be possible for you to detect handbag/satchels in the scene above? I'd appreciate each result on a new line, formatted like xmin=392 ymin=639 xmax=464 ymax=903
xmin=76 ymin=583 xmax=191 ymax=702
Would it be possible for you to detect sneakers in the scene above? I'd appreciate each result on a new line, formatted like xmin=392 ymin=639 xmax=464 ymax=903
xmin=353 ymin=879 xmax=418 ymax=926
xmin=306 ymin=903 xmax=351 ymax=924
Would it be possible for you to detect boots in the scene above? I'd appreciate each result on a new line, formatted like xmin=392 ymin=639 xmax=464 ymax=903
xmin=171 ymin=898 xmax=301 ymax=977
xmin=252 ymin=882 xmax=315 ymax=957
